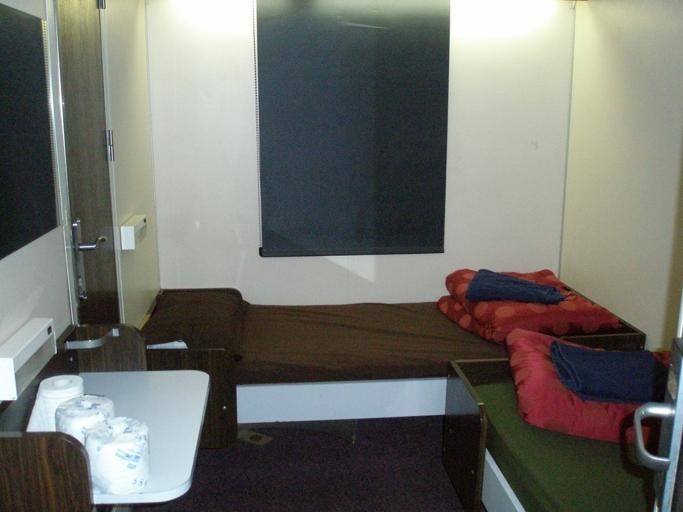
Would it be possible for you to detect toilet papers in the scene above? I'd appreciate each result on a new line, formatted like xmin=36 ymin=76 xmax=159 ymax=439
xmin=83 ymin=417 xmax=150 ymax=496
xmin=25 ymin=374 xmax=85 ymax=433
xmin=55 ymin=394 xmax=116 ymax=447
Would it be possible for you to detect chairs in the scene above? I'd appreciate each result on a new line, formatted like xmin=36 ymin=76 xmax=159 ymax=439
xmin=63 ymin=321 xmax=148 ymax=369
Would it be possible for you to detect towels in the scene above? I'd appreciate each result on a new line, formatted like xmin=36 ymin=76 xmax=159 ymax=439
xmin=464 ymin=268 xmax=566 ymax=306
xmin=550 ymin=340 xmax=668 ymax=402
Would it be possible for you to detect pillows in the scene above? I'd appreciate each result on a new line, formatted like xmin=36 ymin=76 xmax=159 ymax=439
xmin=139 ymin=288 xmax=245 ymax=357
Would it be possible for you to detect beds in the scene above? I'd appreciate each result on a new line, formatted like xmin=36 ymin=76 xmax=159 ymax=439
xmin=137 ymin=280 xmax=647 ymax=424
